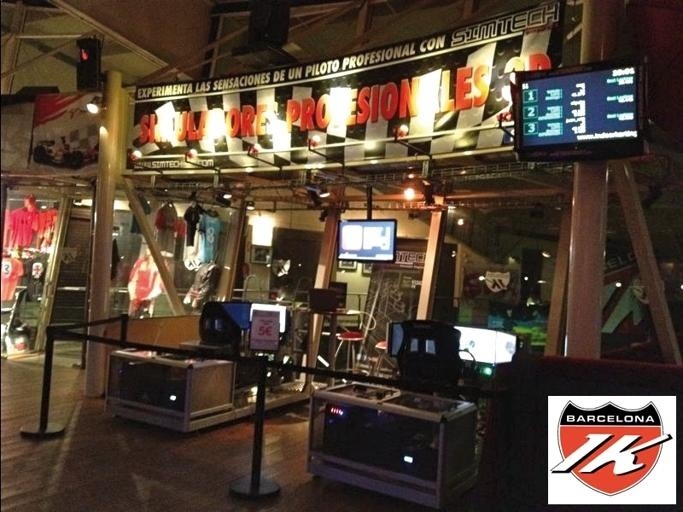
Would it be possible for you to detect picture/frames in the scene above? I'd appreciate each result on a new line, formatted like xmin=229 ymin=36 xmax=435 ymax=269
xmin=250 ymin=245 xmax=273 ymax=265
xmin=337 ymin=260 xmax=373 ymax=277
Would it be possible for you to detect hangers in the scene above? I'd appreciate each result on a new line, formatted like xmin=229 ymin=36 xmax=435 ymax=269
xmin=205 ymin=203 xmax=219 ymax=217
xmin=166 ymin=201 xmax=175 ymax=208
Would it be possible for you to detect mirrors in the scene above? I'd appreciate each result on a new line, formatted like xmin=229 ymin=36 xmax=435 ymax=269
xmin=1 ymin=186 xmax=73 ymax=353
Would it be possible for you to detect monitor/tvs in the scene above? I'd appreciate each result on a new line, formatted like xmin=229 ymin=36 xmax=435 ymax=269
xmin=510 ymin=54 xmax=650 ymax=162
xmin=219 ymin=301 xmax=287 ymax=334
xmin=387 ymin=322 xmax=520 ymax=366
xmin=336 ymin=219 xmax=397 ymax=264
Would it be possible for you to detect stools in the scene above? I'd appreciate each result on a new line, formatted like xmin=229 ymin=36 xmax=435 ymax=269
xmin=327 ymin=333 xmax=399 ymax=397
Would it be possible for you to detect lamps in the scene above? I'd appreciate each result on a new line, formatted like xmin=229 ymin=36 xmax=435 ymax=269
xmin=85 ymin=95 xmax=108 ymax=114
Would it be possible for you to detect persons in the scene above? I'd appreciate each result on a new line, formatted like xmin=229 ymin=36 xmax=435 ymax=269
xmin=126 ymin=244 xmax=162 ymax=319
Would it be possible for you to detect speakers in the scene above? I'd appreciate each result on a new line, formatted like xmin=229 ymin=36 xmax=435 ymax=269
xmin=76 ymin=39 xmax=102 ymax=91
xmin=248 ymin=2 xmax=290 ymax=51
